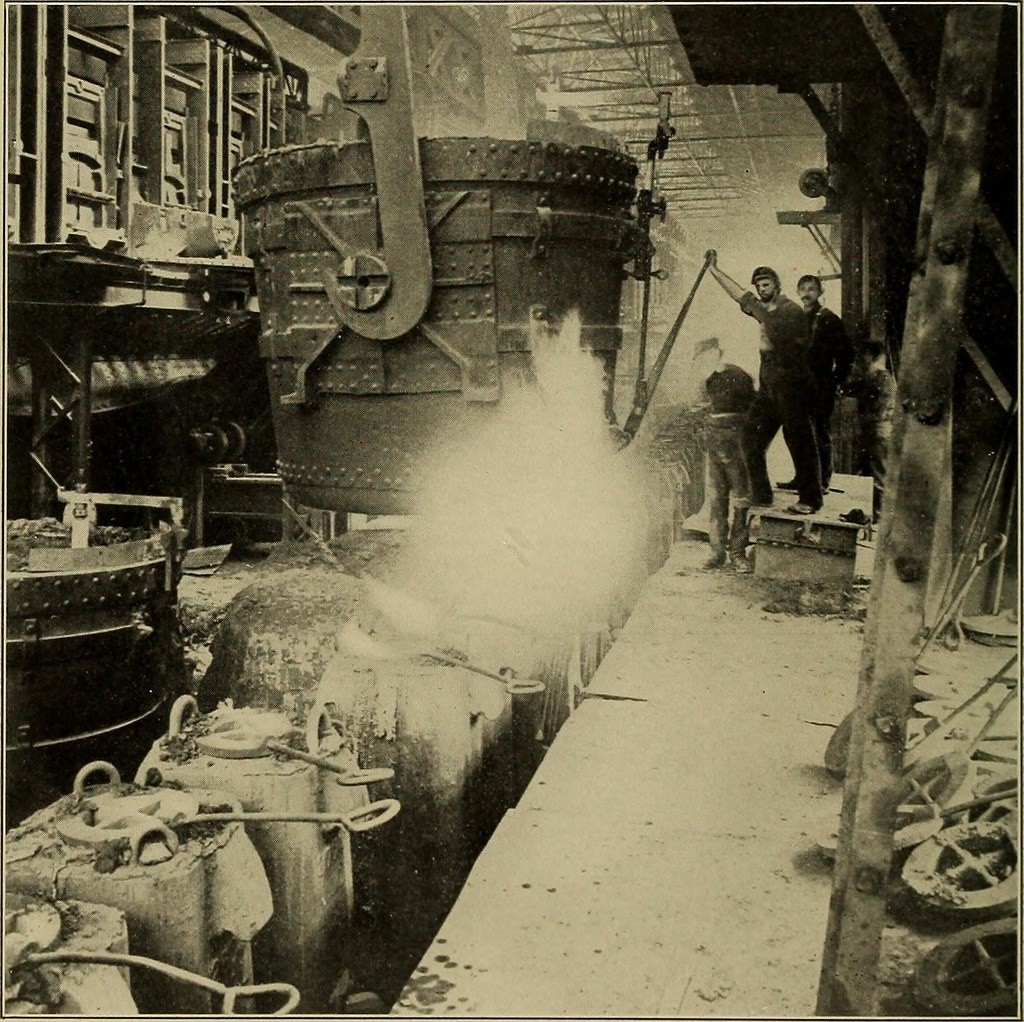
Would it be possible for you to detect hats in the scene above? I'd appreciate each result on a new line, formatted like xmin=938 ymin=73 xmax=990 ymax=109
xmin=751 ymin=266 xmax=777 ymax=285
xmin=693 ymin=337 xmax=719 ymax=360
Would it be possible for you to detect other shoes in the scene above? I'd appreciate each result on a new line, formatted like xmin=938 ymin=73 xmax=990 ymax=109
xmin=731 ymin=556 xmax=751 ymax=573
xmin=788 ymin=501 xmax=815 ymax=514
xmin=750 ymin=498 xmax=772 ymax=507
xmin=776 ymin=477 xmax=798 ymax=490
xmin=700 ymin=557 xmax=724 ymax=572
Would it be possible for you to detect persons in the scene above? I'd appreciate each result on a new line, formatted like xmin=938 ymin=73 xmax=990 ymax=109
xmin=704 ymin=248 xmax=824 ymax=515
xmin=776 ymin=274 xmax=851 ymax=495
xmin=690 ymin=337 xmax=757 ymax=573
xmin=844 ymin=320 xmax=895 ymax=523
